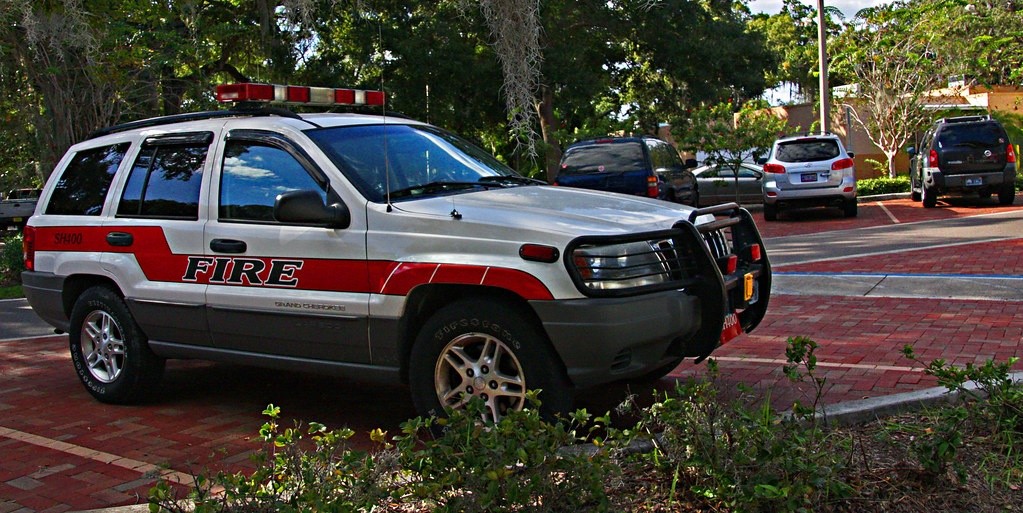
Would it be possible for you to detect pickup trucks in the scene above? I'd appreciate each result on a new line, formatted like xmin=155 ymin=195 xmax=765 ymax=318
xmin=0 ymin=187 xmax=45 ymax=233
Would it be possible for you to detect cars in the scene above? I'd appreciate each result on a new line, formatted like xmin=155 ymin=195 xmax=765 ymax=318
xmin=690 ymin=162 xmax=763 ymax=209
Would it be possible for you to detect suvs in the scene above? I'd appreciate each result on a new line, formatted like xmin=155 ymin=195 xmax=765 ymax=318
xmin=907 ymin=114 xmax=1017 ymax=208
xmin=22 ymin=81 xmax=772 ymax=468
xmin=553 ymin=134 xmax=700 ymax=209
xmin=758 ymin=132 xmax=858 ymax=221
